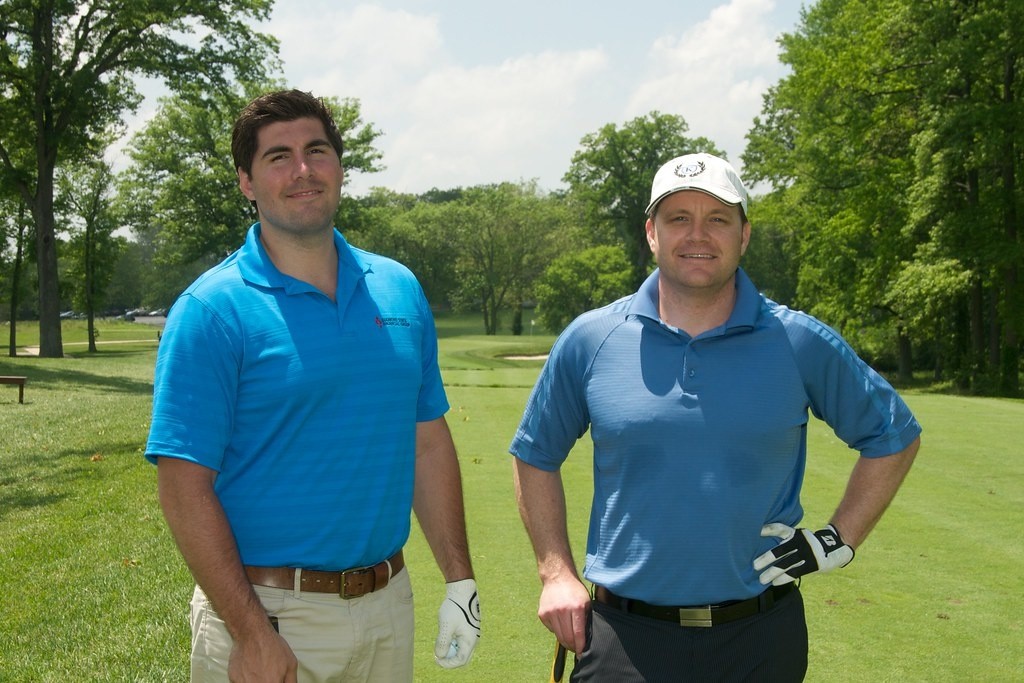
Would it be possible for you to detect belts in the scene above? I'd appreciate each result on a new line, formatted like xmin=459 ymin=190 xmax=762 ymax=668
xmin=593 ymin=584 xmax=777 ymax=628
xmin=241 ymin=549 xmax=405 ymax=600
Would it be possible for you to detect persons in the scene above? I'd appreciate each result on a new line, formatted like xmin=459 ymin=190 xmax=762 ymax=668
xmin=143 ymin=88 xmax=481 ymax=683
xmin=508 ymin=155 xmax=921 ymax=683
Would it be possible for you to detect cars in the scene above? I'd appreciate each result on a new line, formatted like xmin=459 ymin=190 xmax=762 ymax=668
xmin=125 ymin=305 xmax=151 ymax=317
xmin=60 ymin=310 xmax=84 ymax=319
xmin=149 ymin=308 xmax=169 ymax=318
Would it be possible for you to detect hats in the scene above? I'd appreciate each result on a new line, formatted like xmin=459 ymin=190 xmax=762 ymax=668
xmin=644 ymin=152 xmax=749 ymax=222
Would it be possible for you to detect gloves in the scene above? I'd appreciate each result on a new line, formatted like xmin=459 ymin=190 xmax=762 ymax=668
xmin=752 ymin=523 xmax=854 ymax=587
xmin=434 ymin=578 xmax=482 ymax=670
